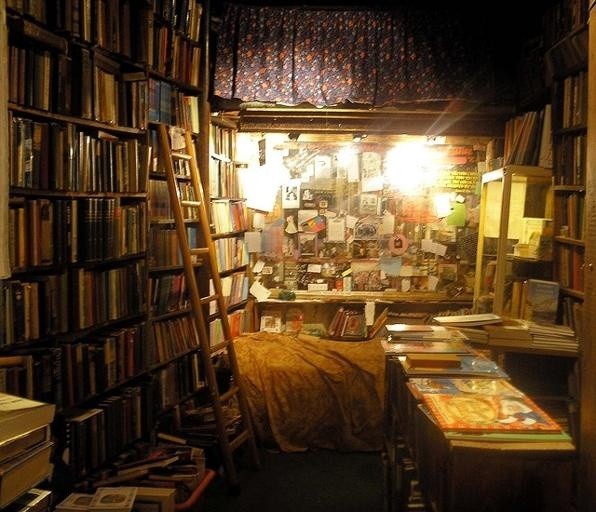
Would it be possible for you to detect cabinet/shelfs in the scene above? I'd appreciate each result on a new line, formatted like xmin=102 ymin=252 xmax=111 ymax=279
xmin=0 ymin=0 xmax=209 ymax=512
xmin=473 ymin=164 xmax=552 ymax=316
xmin=208 ymin=116 xmax=256 ymax=358
xmin=552 ymin=0 xmax=596 ymax=401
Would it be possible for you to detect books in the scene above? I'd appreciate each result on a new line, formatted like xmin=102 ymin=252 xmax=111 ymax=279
xmin=328 ymin=303 xmax=365 ymax=337
xmin=503 ymin=84 xmax=589 ymax=331
xmin=0 ymin=1 xmax=248 ymax=512
xmin=384 ymin=310 xmax=579 ymax=442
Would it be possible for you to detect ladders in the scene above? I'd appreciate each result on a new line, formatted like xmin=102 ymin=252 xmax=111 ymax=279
xmin=159 ymin=124 xmax=262 ymax=486
